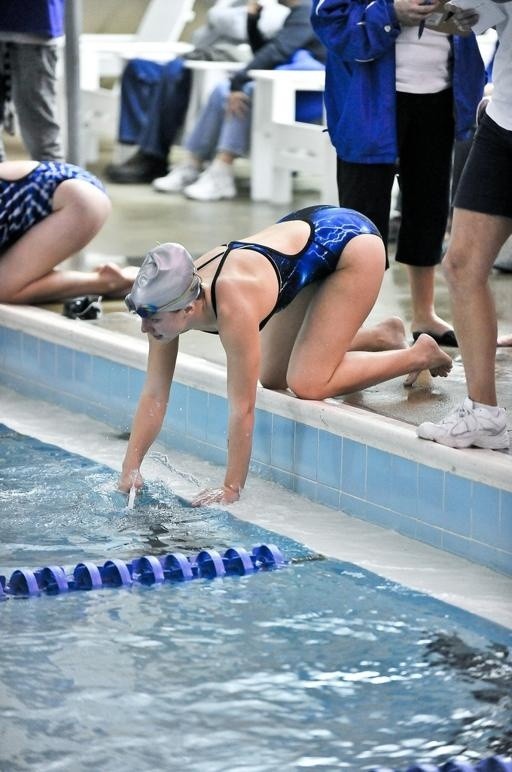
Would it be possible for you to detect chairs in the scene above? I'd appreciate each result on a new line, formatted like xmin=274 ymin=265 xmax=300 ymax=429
xmin=9 ymin=0 xmax=338 ymax=206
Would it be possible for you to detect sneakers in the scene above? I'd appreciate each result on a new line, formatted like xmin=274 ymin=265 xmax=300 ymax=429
xmin=416 ymin=397 xmax=511 ymax=453
xmin=182 ymin=163 xmax=239 ymax=202
xmin=104 ymin=148 xmax=172 ymax=184
xmin=151 ymin=158 xmax=200 ymax=193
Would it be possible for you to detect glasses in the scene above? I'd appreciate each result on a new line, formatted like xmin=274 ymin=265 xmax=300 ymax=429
xmin=63 ymin=294 xmax=102 ymax=320
xmin=125 ymin=268 xmax=198 ymax=319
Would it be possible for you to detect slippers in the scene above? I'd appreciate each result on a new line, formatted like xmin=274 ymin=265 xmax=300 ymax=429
xmin=413 ymin=329 xmax=461 ymax=347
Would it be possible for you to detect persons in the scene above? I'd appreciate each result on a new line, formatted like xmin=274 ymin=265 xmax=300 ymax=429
xmin=310 ymin=0 xmax=488 ymax=347
xmin=153 ymin=0 xmax=328 ymax=201
xmin=0 ymin=159 xmax=141 ymax=304
xmin=0 ymin=0 xmax=64 ymax=162
xmin=105 ymin=0 xmax=291 ymax=184
xmin=118 ymin=205 xmax=453 ymax=508
xmin=418 ymin=0 xmax=512 ymax=449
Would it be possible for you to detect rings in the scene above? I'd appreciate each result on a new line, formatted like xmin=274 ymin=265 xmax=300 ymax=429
xmin=457 ymin=26 xmax=464 ymax=32
xmin=453 ymin=19 xmax=460 ymax=27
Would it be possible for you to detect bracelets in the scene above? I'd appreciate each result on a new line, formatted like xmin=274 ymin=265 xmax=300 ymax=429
xmin=224 ymin=482 xmax=239 ymax=493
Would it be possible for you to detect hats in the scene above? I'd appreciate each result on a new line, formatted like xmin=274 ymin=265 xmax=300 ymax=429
xmin=131 ymin=242 xmax=201 ymax=312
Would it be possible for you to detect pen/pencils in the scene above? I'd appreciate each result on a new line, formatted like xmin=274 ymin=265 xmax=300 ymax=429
xmin=417 ymin=1 xmax=429 ymax=38
xmin=442 ymin=9 xmax=456 ymax=24
